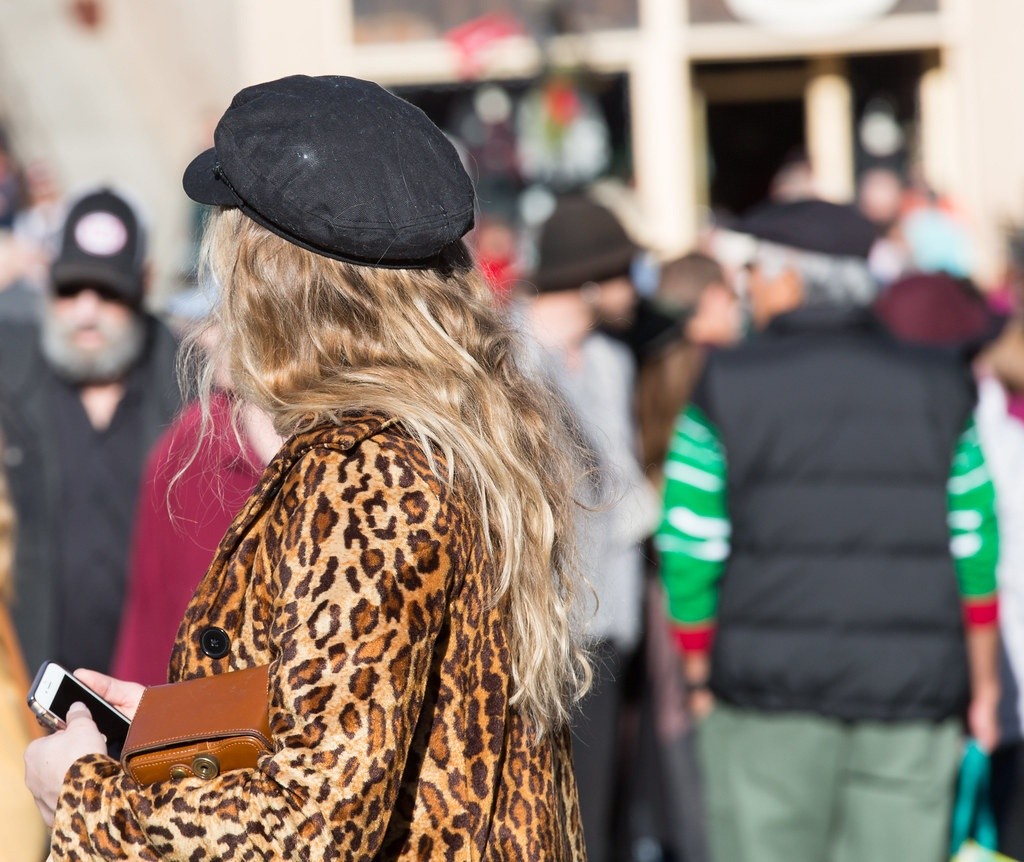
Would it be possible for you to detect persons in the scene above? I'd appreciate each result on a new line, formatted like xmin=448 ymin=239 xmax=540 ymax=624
xmin=24 ymin=74 xmax=621 ymax=862
xmin=462 ymin=163 xmax=1024 ymax=862
xmin=0 ymin=120 xmax=289 ymax=862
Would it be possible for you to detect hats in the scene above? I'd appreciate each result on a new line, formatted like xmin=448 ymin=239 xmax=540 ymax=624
xmin=50 ymin=186 xmax=148 ymax=294
xmin=531 ymin=197 xmax=645 ymax=292
xmin=720 ymin=198 xmax=877 ymax=260
xmin=181 ymin=73 xmax=476 ymax=269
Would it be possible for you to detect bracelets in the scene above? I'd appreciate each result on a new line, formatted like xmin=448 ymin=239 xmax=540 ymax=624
xmin=684 ymin=678 xmax=711 ymax=693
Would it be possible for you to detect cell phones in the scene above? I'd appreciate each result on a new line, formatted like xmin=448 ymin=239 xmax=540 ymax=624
xmin=25 ymin=660 xmax=133 ymax=763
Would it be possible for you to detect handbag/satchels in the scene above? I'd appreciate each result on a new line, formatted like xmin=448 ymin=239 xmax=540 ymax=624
xmin=118 ymin=659 xmax=275 ymax=792
